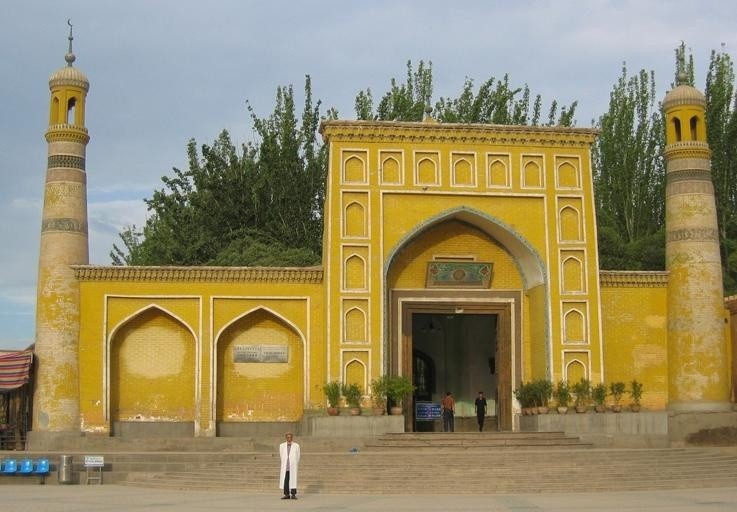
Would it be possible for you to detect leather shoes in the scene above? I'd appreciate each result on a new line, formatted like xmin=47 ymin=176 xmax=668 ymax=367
xmin=280 ymin=495 xmax=289 ymax=498
xmin=292 ymin=495 xmax=297 ymax=499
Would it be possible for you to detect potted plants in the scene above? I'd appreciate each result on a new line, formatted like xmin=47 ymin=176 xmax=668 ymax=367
xmin=513 ymin=378 xmax=643 ymax=415
xmin=322 ymin=373 xmax=417 ymax=415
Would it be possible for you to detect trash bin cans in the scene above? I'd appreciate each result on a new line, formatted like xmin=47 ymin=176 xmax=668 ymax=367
xmin=58 ymin=455 xmax=73 ymax=484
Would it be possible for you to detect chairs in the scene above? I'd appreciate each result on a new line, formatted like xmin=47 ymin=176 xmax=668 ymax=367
xmin=1 ymin=457 xmax=50 ymax=484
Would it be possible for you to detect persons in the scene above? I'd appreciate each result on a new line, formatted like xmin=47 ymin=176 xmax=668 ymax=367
xmin=440 ymin=391 xmax=455 ymax=432
xmin=473 ymin=391 xmax=487 ymax=432
xmin=277 ymin=432 xmax=301 ymax=500
